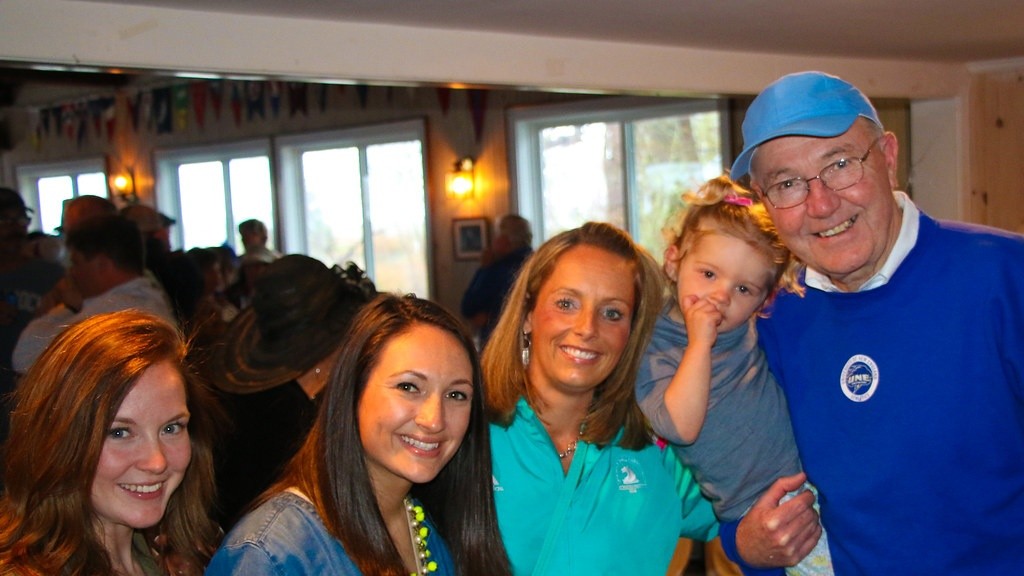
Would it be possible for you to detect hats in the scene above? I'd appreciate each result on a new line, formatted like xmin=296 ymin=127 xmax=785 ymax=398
xmin=1 ymin=188 xmax=34 ymax=213
xmin=726 ymin=70 xmax=883 ymax=182
xmin=206 ymin=253 xmax=386 ymax=395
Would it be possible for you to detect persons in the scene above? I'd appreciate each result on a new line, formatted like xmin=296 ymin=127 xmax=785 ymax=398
xmin=481 ymin=221 xmax=720 ymax=576
xmin=730 ymin=71 xmax=1024 ymax=576
xmin=0 ymin=307 xmax=222 ymax=576
xmin=634 ymin=176 xmax=835 ymax=576
xmin=0 ymin=187 xmax=533 ymax=369
xmin=202 ymin=293 xmax=516 ymax=576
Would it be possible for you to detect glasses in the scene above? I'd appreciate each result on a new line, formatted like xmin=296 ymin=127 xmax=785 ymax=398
xmin=755 ymin=137 xmax=883 ymax=210
xmin=1 ymin=216 xmax=31 ymax=227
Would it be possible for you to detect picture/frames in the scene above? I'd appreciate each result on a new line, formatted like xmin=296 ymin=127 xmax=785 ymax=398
xmin=451 ymin=216 xmax=491 ymax=261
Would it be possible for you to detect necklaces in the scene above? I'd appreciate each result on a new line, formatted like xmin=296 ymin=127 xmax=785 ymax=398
xmin=558 ymin=422 xmax=583 ymax=461
xmin=404 ymin=495 xmax=438 ymax=576
